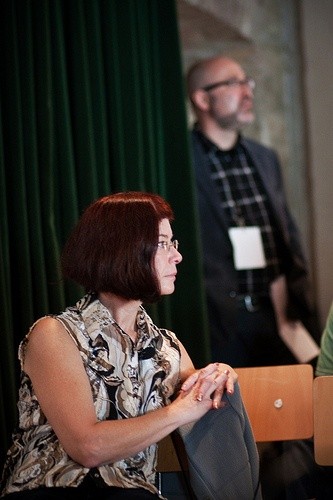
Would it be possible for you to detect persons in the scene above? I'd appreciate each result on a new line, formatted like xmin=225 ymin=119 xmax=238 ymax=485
xmin=185 ymin=59 xmax=315 ymax=500
xmin=0 ymin=193 xmax=240 ymax=500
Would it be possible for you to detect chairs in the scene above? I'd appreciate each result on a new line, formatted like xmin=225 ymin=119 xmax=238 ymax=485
xmin=312 ymin=376 xmax=333 ymax=466
xmin=155 ymin=430 xmax=191 ymax=493
xmin=233 ymin=364 xmax=314 ymax=442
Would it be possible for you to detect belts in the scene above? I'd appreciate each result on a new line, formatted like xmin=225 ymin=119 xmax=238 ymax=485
xmin=236 ymin=293 xmax=270 ymax=310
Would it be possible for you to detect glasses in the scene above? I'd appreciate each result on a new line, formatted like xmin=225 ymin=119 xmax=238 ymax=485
xmin=204 ymin=77 xmax=255 ymax=91
xmin=158 ymin=240 xmax=180 ymax=253
